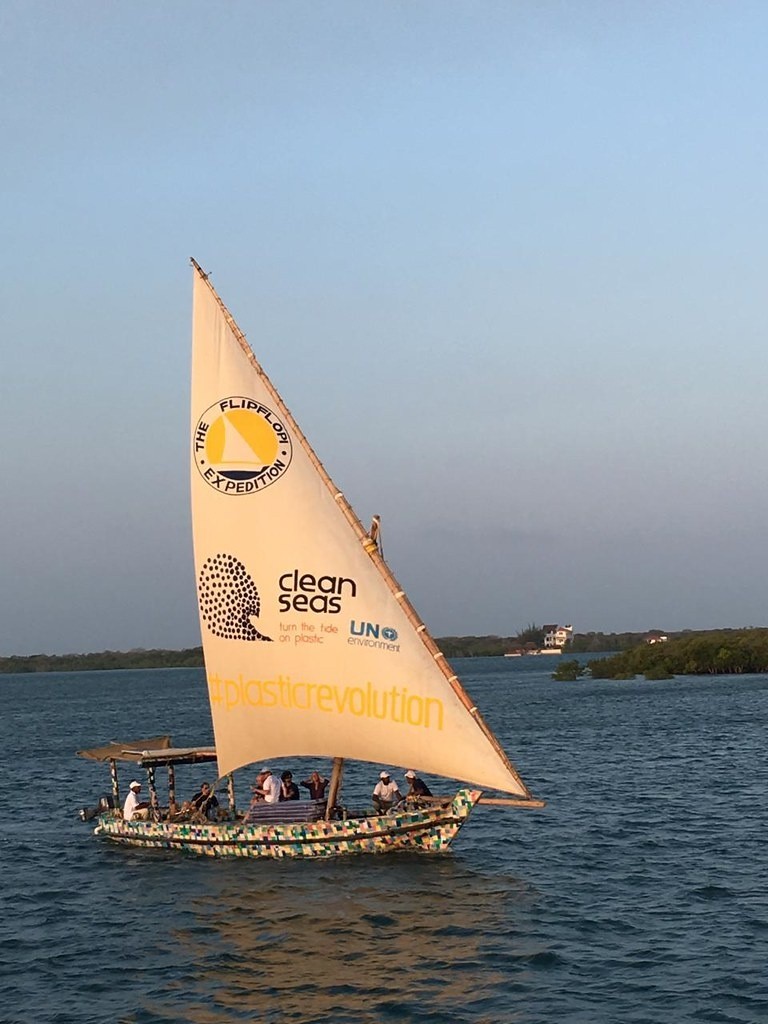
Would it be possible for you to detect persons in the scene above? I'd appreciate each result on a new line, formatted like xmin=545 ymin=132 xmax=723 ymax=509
xmin=122 ymin=778 xmax=149 ymax=823
xmin=189 ymin=780 xmax=220 ymax=822
xmin=240 ymin=766 xmax=299 ymax=825
xmin=371 ymin=770 xmax=400 ymax=816
xmin=301 ymin=770 xmax=331 ymax=799
xmin=402 ymin=769 xmax=429 ymax=796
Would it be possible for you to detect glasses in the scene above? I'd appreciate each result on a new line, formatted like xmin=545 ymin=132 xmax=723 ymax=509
xmin=202 ymin=787 xmax=209 ymax=790
xmin=285 ymin=776 xmax=292 ymax=779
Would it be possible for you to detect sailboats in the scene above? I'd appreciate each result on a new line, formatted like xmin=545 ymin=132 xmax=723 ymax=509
xmin=72 ymin=261 xmax=546 ymax=859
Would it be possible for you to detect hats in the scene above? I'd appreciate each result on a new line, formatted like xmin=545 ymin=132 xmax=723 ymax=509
xmin=404 ymin=771 xmax=416 ymax=779
xmin=259 ymin=766 xmax=273 ymax=774
xmin=380 ymin=770 xmax=392 ymax=779
xmin=129 ymin=780 xmax=142 ymax=789
